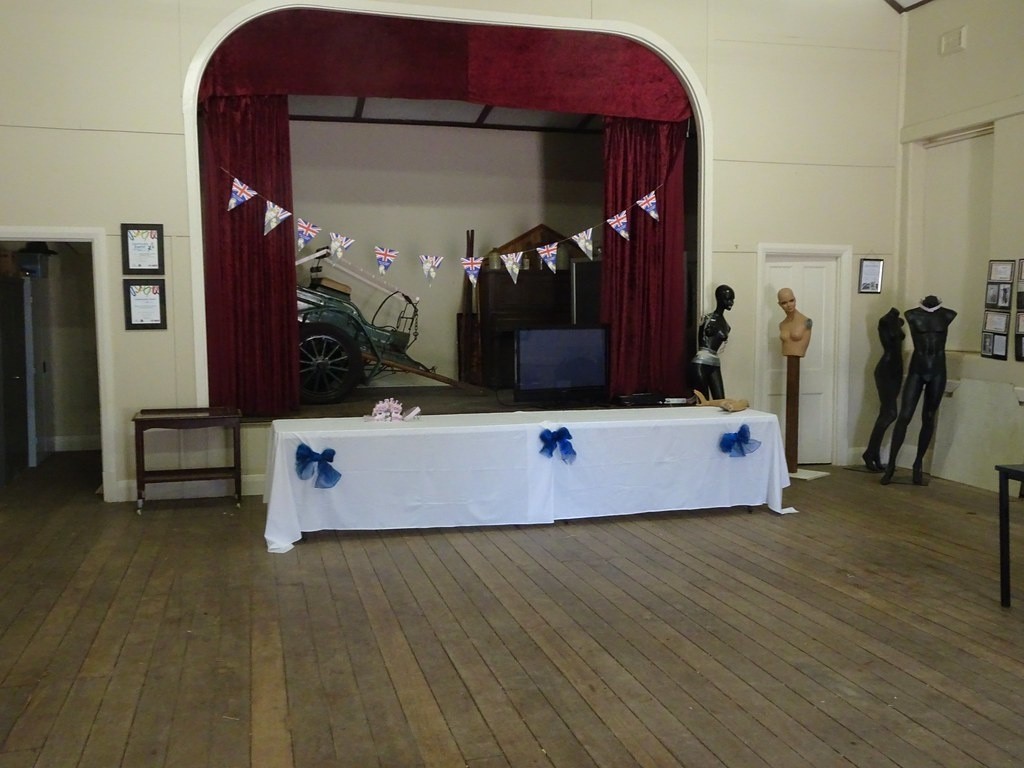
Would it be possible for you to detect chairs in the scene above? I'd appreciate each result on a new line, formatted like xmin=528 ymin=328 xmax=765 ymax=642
xmin=309 ymin=246 xmax=352 ymax=302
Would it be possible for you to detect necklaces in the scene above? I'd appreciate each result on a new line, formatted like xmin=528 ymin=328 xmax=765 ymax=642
xmin=919 ymin=301 xmax=943 ymax=312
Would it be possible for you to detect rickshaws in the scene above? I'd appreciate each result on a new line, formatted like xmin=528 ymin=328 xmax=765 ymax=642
xmin=297 ymin=286 xmax=489 ymax=405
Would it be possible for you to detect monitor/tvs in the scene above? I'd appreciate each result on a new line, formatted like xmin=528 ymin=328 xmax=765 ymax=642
xmin=514 ymin=324 xmax=610 ymax=408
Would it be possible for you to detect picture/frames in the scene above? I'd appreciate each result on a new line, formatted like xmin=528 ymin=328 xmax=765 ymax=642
xmin=1015 ymin=312 xmax=1024 ymax=335
xmin=982 ymin=310 xmax=1011 ymax=335
xmin=1017 ymin=259 xmax=1024 ymax=283
xmin=987 ymin=259 xmax=1015 ymax=283
xmin=858 ymin=257 xmax=884 ymax=294
xmin=122 ymin=278 xmax=168 ymax=329
xmin=120 ymin=223 xmax=165 ymax=275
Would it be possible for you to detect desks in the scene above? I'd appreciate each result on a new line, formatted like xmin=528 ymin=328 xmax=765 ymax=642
xmin=994 ymin=464 xmax=1024 ymax=606
xmin=520 ymin=407 xmax=800 ymax=526
xmin=261 ymin=411 xmax=555 ymax=554
xmin=130 ymin=407 xmax=243 ymax=515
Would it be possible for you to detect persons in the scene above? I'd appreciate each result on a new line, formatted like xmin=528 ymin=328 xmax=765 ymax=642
xmin=693 ymin=284 xmax=735 ymax=400
xmin=880 ymin=296 xmax=958 ymax=486
xmin=862 ymin=307 xmax=906 ymax=471
xmin=694 ymin=390 xmax=749 ymax=412
xmin=777 ymin=288 xmax=813 ymax=357
